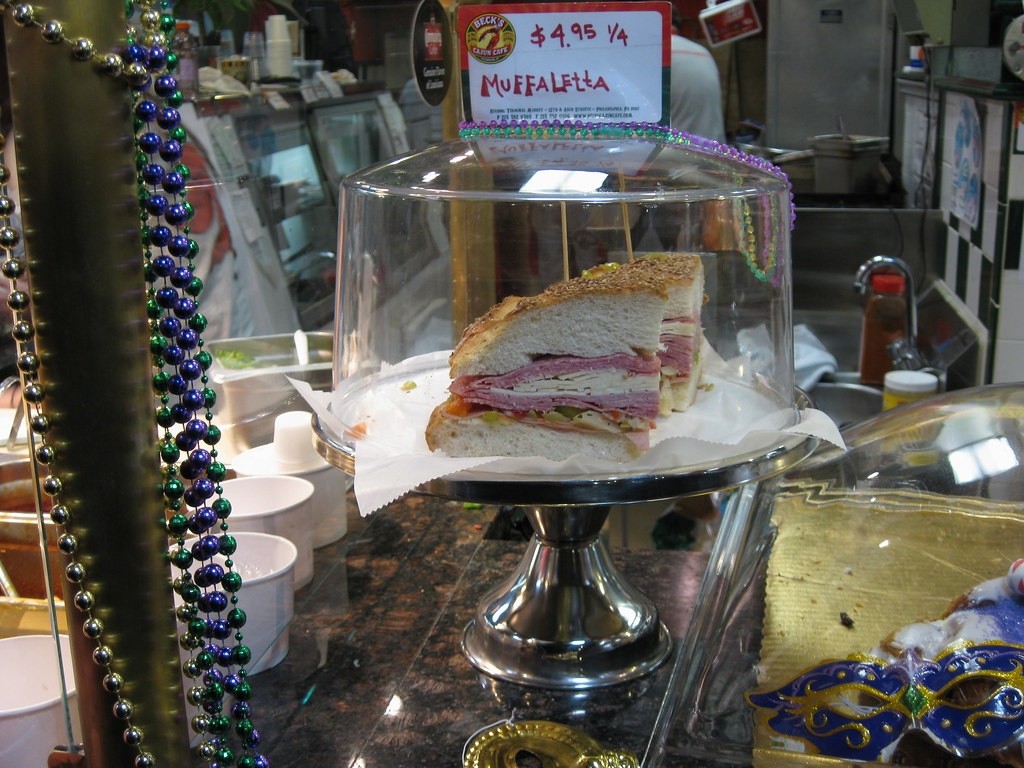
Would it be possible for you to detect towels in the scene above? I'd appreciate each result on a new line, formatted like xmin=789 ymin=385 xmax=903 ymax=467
xmin=735 ymin=324 xmax=837 ymax=394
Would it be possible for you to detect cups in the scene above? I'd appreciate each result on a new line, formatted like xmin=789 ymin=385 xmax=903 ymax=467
xmin=242 ymin=32 xmax=267 ymax=82
xmin=213 ymin=29 xmax=235 ymax=72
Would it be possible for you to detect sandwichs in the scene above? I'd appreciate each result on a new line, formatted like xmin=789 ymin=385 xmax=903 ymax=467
xmin=620 ymin=252 xmax=703 ymax=412
xmin=426 ymin=256 xmax=661 ymax=460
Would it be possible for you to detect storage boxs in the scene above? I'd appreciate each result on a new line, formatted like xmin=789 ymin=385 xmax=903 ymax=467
xmin=811 ymin=133 xmax=890 ymax=196
xmin=928 ymin=42 xmax=1005 ymax=85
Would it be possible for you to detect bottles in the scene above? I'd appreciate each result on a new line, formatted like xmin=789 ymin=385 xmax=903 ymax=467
xmin=882 ymin=369 xmax=938 ymax=412
xmin=858 ymin=273 xmax=909 ymax=386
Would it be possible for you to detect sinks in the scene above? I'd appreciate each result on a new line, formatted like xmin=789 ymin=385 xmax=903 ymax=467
xmin=780 ymin=310 xmax=864 ymax=381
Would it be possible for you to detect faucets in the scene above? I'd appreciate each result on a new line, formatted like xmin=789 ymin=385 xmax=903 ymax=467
xmin=853 ymin=254 xmax=920 ymax=372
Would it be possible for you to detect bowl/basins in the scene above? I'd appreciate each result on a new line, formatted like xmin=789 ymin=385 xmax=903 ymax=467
xmin=183 ymin=474 xmax=316 ymax=592
xmin=0 ymin=633 xmax=86 ymax=768
xmin=168 ymin=529 xmax=296 ymax=677
xmin=231 ymin=439 xmax=348 ymax=552
xmin=264 ymin=15 xmax=295 ymax=77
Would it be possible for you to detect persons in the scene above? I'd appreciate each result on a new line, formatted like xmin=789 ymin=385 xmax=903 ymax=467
xmin=646 ymin=32 xmax=726 ymax=166
